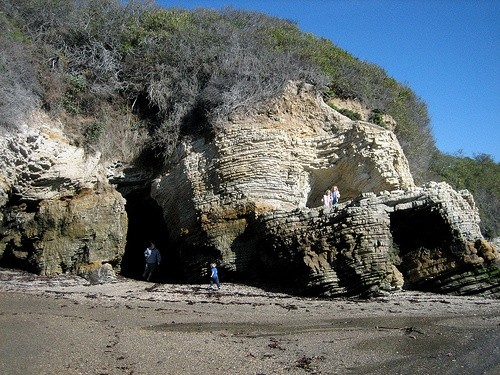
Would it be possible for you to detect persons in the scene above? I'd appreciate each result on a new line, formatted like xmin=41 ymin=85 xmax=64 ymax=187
xmin=210 ymin=262 xmax=221 ymax=290
xmin=321 ymin=190 xmax=333 ymax=208
xmin=330 ymin=185 xmax=341 ymax=205
xmin=143 ymin=240 xmax=162 ymax=281
